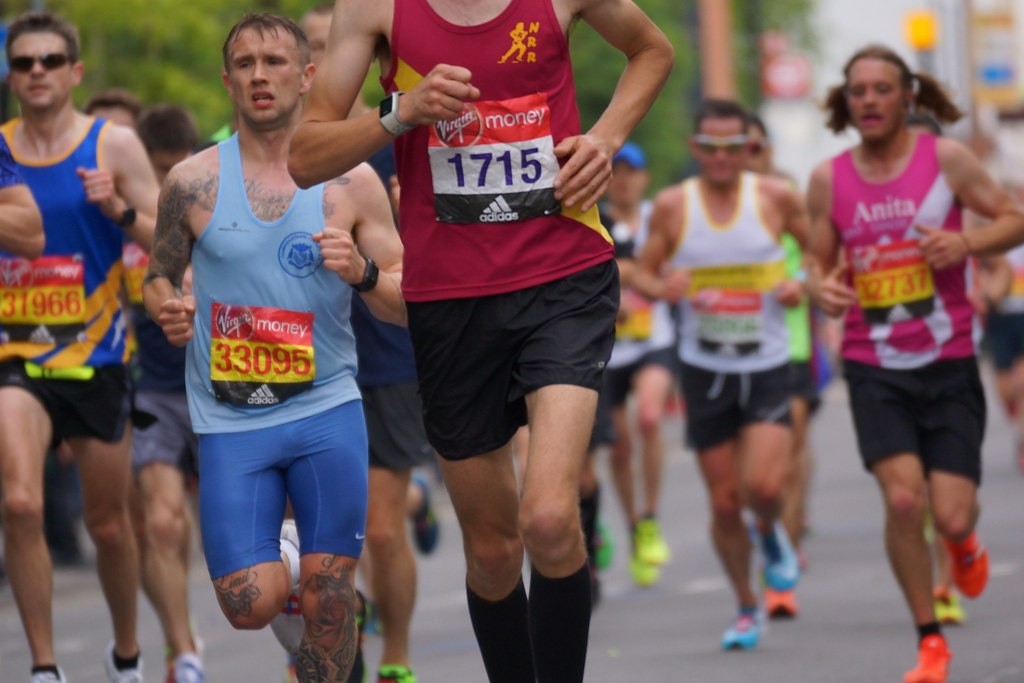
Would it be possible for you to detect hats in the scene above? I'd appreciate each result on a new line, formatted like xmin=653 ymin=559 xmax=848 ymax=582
xmin=614 ymin=142 xmax=646 ymax=168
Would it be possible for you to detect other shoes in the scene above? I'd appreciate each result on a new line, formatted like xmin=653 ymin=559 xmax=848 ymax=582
xmin=376 ymin=665 xmax=416 ymax=683
xmin=166 ymin=636 xmax=206 ymax=683
xmin=103 ymin=639 xmax=144 ymax=683
xmin=765 ymin=589 xmax=798 ymax=619
xmin=630 ymin=518 xmax=666 ymax=589
xmin=409 ymin=479 xmax=437 ymax=552
xmin=763 ymin=521 xmax=801 ymax=592
xmin=596 ymin=514 xmax=615 ymax=572
xmin=31 ymin=663 xmax=66 ymax=683
xmin=346 ymin=589 xmax=369 ymax=682
xmin=721 ymin=601 xmax=763 ymax=650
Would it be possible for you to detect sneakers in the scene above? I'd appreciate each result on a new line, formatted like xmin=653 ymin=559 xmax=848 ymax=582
xmin=270 ymin=519 xmax=305 ymax=654
xmin=945 ymin=529 xmax=988 ymax=599
xmin=934 ymin=595 xmax=964 ymax=624
xmin=904 ymin=635 xmax=952 ymax=683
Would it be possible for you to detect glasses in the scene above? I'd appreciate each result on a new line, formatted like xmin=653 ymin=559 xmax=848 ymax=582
xmin=740 ymin=137 xmax=767 ymax=156
xmin=697 ymin=134 xmax=746 ymax=154
xmin=11 ymin=53 xmax=72 ymax=72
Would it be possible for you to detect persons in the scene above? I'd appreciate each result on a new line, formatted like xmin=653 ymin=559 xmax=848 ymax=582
xmin=0 ymin=0 xmax=1024 ymax=683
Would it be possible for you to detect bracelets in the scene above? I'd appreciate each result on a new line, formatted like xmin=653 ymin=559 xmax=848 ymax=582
xmin=959 ymin=232 xmax=972 ymax=253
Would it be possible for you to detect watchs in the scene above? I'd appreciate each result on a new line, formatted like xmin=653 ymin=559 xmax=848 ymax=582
xmin=348 ymin=256 xmax=379 ymax=293
xmin=120 ymin=208 xmax=136 ymax=226
xmin=379 ymin=91 xmax=413 ymax=136
